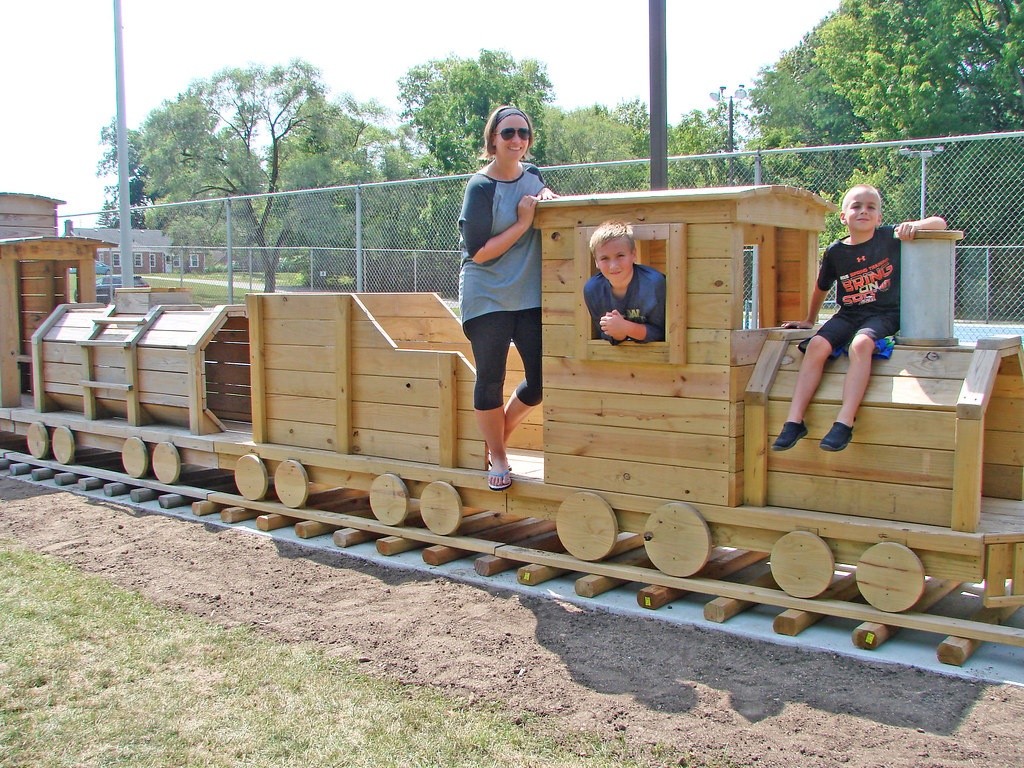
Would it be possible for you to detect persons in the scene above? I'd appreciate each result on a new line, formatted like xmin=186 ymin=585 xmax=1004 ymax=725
xmin=771 ymin=185 xmax=946 ymax=451
xmin=584 ymin=219 xmax=666 ymax=343
xmin=459 ymin=107 xmax=560 ymax=490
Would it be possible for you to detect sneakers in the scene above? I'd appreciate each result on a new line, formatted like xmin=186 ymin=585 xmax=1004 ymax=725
xmin=819 ymin=422 xmax=854 ymax=451
xmin=771 ymin=420 xmax=808 ymax=450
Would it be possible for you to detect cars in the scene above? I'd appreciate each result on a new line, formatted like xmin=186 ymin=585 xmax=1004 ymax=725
xmin=69 ymin=260 xmax=112 ymax=275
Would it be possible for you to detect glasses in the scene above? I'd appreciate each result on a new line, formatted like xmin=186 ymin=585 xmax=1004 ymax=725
xmin=492 ymin=127 xmax=531 ymax=140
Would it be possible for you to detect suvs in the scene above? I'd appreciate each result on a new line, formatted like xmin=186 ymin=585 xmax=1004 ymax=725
xmin=74 ymin=275 xmax=150 ymax=304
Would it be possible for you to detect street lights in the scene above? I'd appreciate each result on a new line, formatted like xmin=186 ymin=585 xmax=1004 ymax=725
xmin=709 ymin=83 xmax=747 ymax=186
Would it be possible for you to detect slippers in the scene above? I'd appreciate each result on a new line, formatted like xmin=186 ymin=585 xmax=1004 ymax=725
xmin=488 ymin=470 xmax=512 ymax=490
xmin=489 ymin=460 xmax=512 ymax=471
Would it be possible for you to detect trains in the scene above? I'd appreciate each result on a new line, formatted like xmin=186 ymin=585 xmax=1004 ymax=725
xmin=1 ymin=183 xmax=1023 ymax=666
